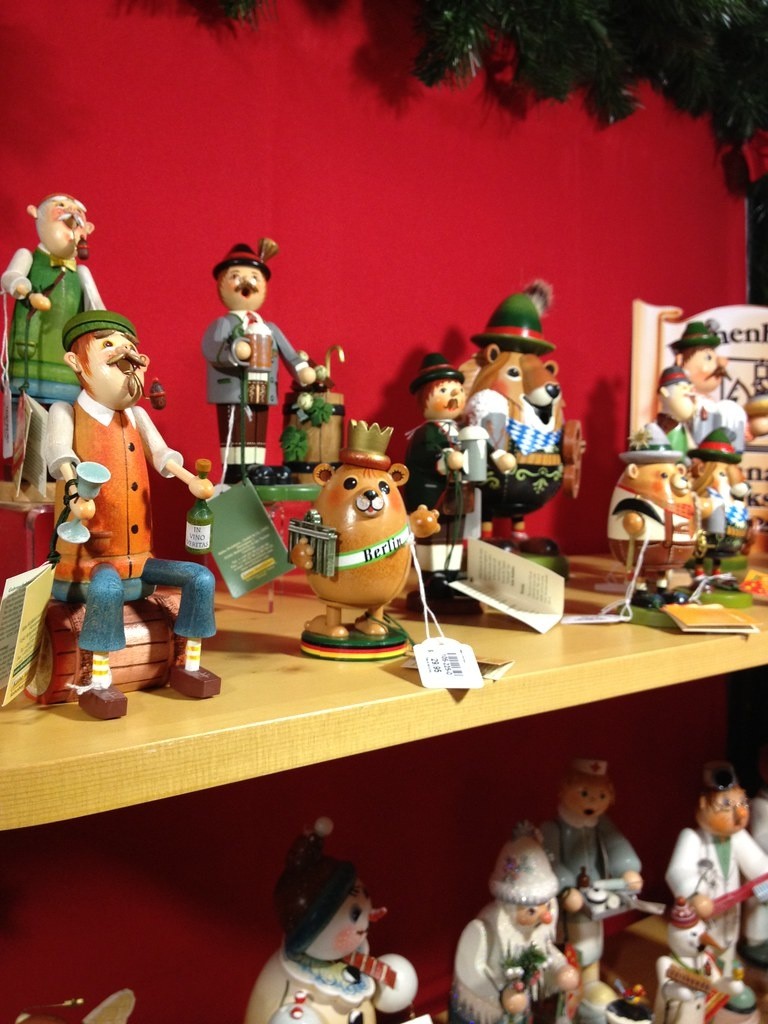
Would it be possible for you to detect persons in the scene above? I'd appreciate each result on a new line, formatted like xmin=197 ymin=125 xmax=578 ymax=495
xmin=631 ymin=323 xmax=741 ymax=606
xmin=1 ymin=194 xmax=222 ymax=720
xmin=245 ymin=760 xmax=768 ymax=1024
xmin=201 ymin=243 xmax=316 ymax=485
xmin=405 ymin=355 xmax=518 ymax=583
